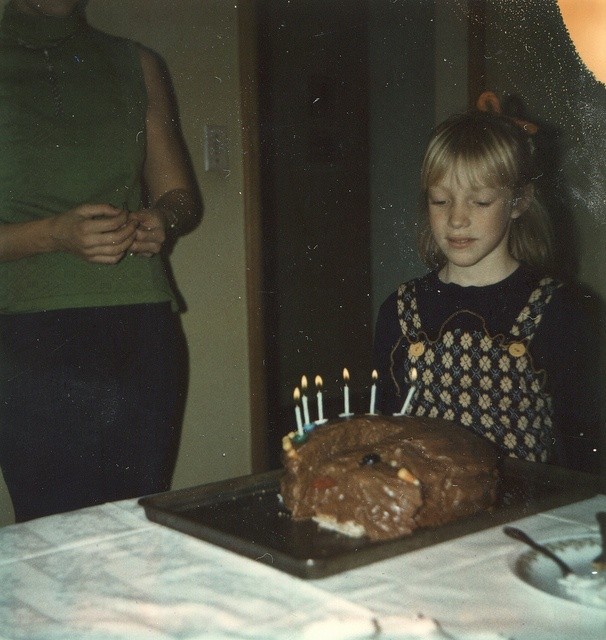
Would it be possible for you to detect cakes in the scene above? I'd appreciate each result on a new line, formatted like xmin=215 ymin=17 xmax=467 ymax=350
xmin=281 ymin=414 xmax=498 ymax=542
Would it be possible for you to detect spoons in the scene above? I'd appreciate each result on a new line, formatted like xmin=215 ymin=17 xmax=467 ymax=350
xmin=501 ymin=525 xmax=575 ymax=579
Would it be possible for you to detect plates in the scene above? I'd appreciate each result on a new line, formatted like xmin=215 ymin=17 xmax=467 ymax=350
xmin=501 ymin=533 xmax=606 ymax=616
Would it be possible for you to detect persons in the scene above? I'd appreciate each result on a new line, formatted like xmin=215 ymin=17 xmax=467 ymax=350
xmin=372 ymin=108 xmax=606 ymax=468
xmin=0 ymin=0 xmax=204 ymax=524
